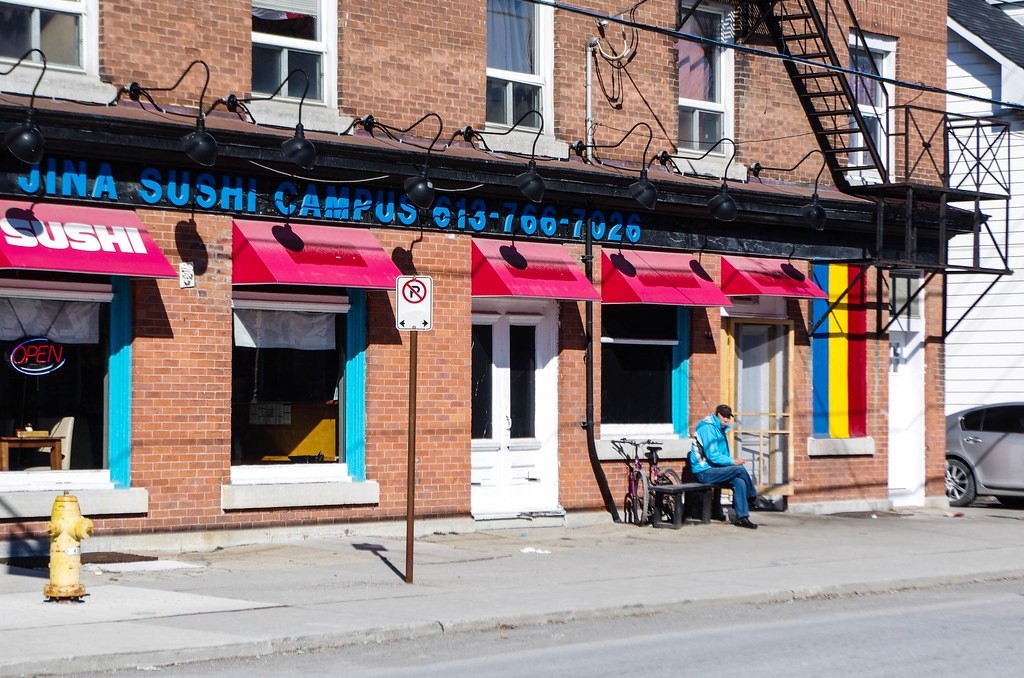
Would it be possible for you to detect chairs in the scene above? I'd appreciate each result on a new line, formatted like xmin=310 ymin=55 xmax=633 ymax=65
xmin=28 ymin=415 xmax=74 ymax=472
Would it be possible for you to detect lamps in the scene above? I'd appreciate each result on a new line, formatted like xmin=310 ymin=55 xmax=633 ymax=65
xmin=205 ymin=68 xmax=314 ymax=171
xmin=0 ymin=49 xmax=48 ymax=166
xmin=647 ymin=137 xmax=738 ymax=223
xmin=341 ymin=110 xmax=444 ymax=209
xmin=110 ymin=59 xmax=218 ymax=168
xmin=749 ymin=150 xmax=830 ymax=233
xmin=448 ymin=111 xmax=547 ymax=204
xmin=567 ymin=122 xmax=658 ymax=211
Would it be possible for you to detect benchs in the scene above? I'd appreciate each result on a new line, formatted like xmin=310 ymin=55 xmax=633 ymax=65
xmin=648 ymin=481 xmax=716 ymax=530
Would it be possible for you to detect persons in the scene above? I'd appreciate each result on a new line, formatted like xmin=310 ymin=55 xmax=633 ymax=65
xmin=691 ymin=404 xmax=767 ymax=529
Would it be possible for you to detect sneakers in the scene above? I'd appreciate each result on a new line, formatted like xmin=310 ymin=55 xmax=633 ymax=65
xmin=748 ymin=497 xmax=765 ymax=509
xmin=735 ymin=517 xmax=757 ymax=528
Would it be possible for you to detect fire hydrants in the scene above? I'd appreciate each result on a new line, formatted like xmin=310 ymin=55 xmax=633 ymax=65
xmin=45 ymin=492 xmax=95 ymax=602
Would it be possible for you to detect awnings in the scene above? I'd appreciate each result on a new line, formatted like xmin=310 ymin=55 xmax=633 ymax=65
xmin=721 ymin=255 xmax=829 ymax=298
xmin=233 ymin=219 xmax=403 ymax=290
xmin=0 ymin=199 xmax=178 ymax=277
xmin=472 ymin=239 xmax=602 ymax=301
xmin=601 ymin=248 xmax=732 ymax=306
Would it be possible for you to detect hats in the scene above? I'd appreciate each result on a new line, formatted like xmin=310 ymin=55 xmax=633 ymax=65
xmin=716 ymin=405 xmax=734 ymax=418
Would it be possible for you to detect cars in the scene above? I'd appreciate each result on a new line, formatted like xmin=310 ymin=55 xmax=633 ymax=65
xmin=945 ymin=401 xmax=1024 ymax=509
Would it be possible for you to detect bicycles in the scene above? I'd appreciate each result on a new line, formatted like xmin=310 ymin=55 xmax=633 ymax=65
xmin=611 ymin=438 xmax=684 ymax=526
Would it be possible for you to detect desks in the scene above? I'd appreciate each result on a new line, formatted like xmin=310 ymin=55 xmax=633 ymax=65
xmin=0 ymin=437 xmax=67 ymax=471
